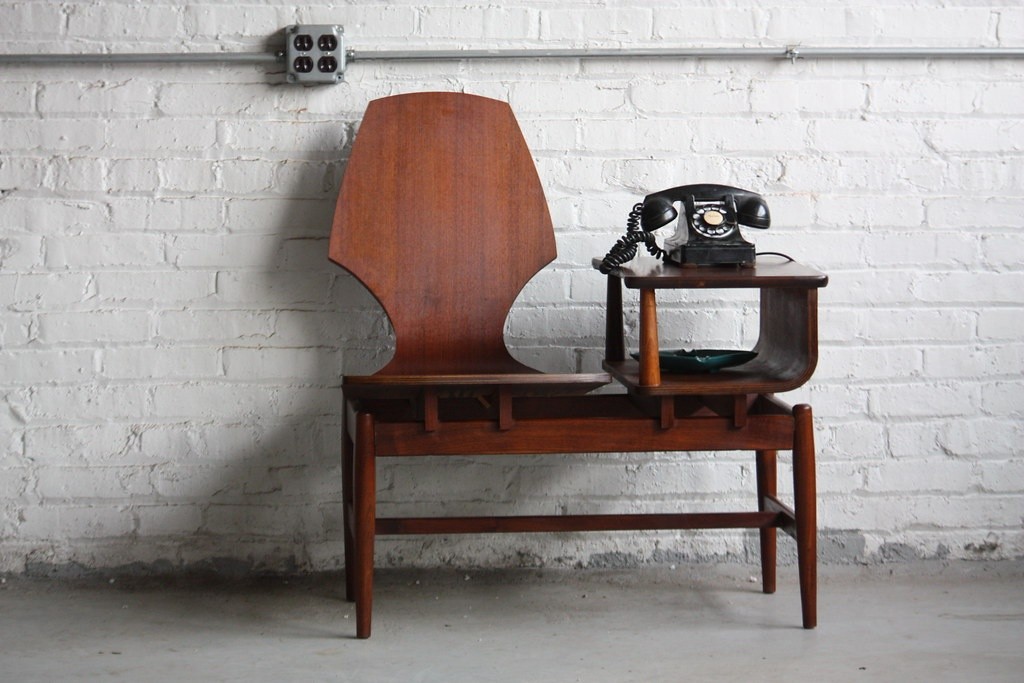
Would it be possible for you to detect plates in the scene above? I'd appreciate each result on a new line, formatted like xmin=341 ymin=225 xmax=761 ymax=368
xmin=630 ymin=350 xmax=759 ymax=375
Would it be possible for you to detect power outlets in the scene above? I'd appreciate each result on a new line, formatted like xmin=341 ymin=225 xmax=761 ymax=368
xmin=285 ymin=24 xmax=345 ymax=85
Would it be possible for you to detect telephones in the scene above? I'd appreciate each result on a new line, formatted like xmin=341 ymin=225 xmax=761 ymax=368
xmin=640 ymin=182 xmax=771 ymax=270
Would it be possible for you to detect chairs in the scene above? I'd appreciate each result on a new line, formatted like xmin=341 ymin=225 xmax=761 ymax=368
xmin=328 ymin=91 xmax=612 ymax=420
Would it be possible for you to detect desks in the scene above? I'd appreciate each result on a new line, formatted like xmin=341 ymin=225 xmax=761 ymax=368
xmin=340 ymin=256 xmax=829 ymax=639
